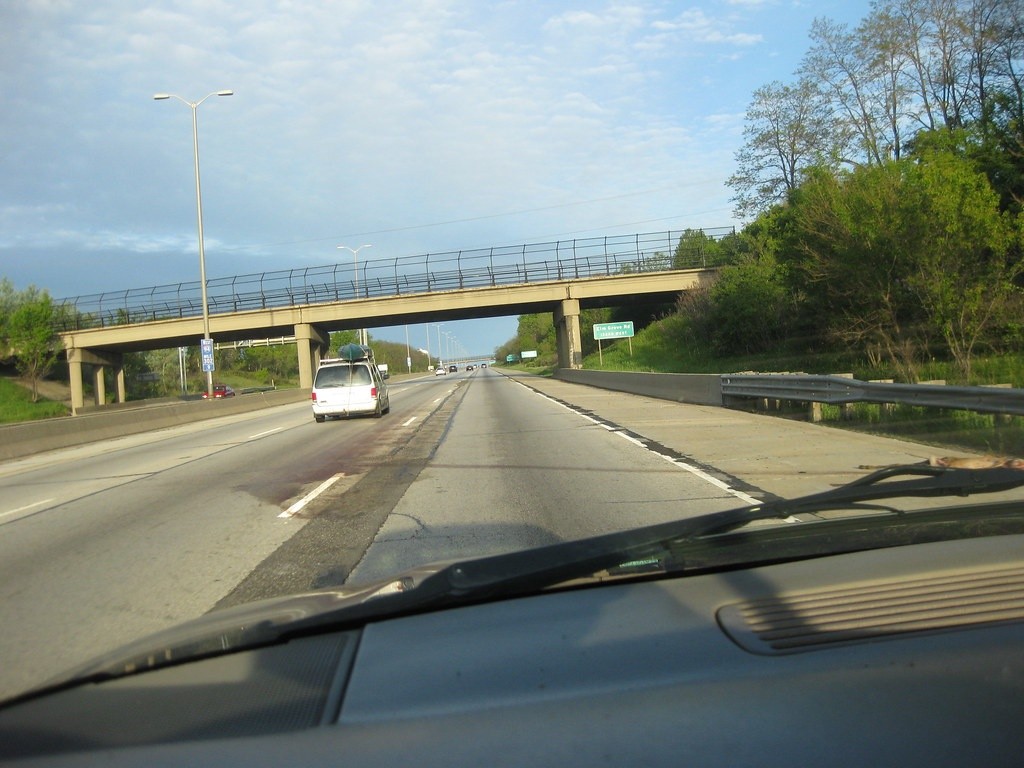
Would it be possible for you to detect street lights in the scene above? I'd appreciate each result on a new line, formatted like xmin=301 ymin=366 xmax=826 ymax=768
xmin=337 ymin=244 xmax=373 ymax=345
xmin=154 ymin=90 xmax=233 ymax=398
xmin=441 ymin=330 xmax=451 ymax=365
xmin=431 ymin=324 xmax=444 ymax=367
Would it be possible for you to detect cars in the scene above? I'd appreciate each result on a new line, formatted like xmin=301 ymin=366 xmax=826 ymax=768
xmin=466 ymin=365 xmax=473 ymax=371
xmin=448 ymin=366 xmax=458 ymax=373
xmin=378 ymin=370 xmax=389 ymax=379
xmin=435 ymin=367 xmax=446 ymax=376
xmin=481 ymin=364 xmax=487 ymax=368
xmin=202 ymin=384 xmax=236 ymax=399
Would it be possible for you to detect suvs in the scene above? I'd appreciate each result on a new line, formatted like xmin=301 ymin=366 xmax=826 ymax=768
xmin=311 ymin=357 xmax=390 ymax=422
